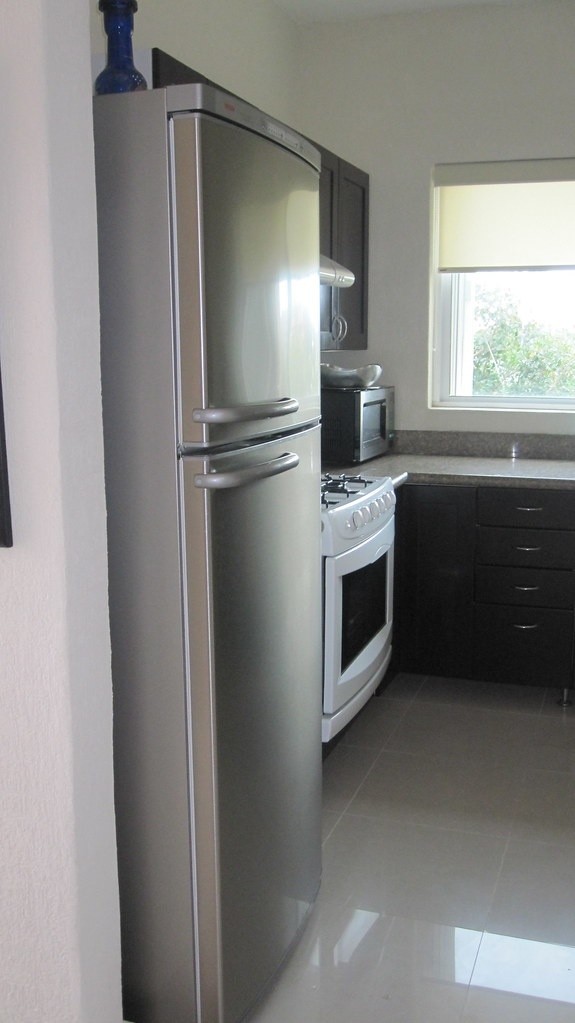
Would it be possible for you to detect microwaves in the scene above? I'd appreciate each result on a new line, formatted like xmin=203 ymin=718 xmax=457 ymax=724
xmin=317 ymin=382 xmax=396 ymax=466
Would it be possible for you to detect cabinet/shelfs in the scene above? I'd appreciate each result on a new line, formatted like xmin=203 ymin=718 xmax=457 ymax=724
xmin=150 ymin=47 xmax=375 ymax=353
xmin=400 ymin=485 xmax=575 ymax=702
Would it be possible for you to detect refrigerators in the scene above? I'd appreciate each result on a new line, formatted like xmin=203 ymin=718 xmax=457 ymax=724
xmin=87 ymin=84 xmax=326 ymax=1021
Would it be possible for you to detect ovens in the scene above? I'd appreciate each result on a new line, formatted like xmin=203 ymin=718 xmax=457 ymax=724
xmin=321 ymin=518 xmax=395 ymax=746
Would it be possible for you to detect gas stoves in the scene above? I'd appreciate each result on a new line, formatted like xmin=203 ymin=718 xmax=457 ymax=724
xmin=320 ymin=468 xmax=396 ymax=557
